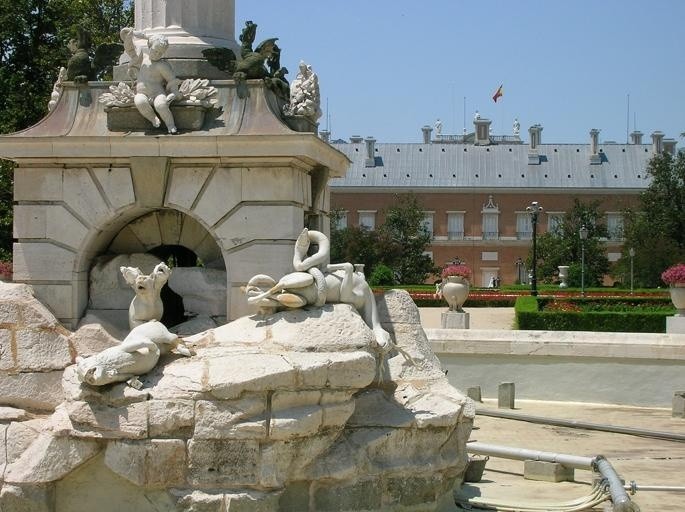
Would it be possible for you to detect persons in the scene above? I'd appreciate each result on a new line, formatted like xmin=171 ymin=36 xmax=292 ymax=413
xmin=488 ymin=276 xmax=500 ymax=288
xmin=434 ymin=276 xmax=443 ymax=298
xmin=120 ymin=26 xmax=182 ymax=136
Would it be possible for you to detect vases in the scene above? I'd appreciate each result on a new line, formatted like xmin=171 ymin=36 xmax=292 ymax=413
xmin=442 ymin=276 xmax=470 ymax=313
xmin=671 ymin=284 xmax=685 ymax=317
xmin=558 ymin=265 xmax=569 ymax=278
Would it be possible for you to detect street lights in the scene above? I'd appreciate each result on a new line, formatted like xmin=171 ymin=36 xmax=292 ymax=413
xmin=514 ymin=259 xmax=525 ymax=285
xmin=525 ymin=202 xmax=544 ymax=296
xmin=627 ymin=246 xmax=636 ymax=293
xmin=452 ymin=257 xmax=460 ymax=265
xmin=578 ymin=225 xmax=589 ymax=293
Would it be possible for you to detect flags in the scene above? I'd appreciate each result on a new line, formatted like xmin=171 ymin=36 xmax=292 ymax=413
xmin=493 ymin=84 xmax=501 ymax=102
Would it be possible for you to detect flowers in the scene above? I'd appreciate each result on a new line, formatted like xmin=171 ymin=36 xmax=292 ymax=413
xmin=441 ymin=266 xmax=473 ymax=279
xmin=661 ymin=264 xmax=685 ymax=283
